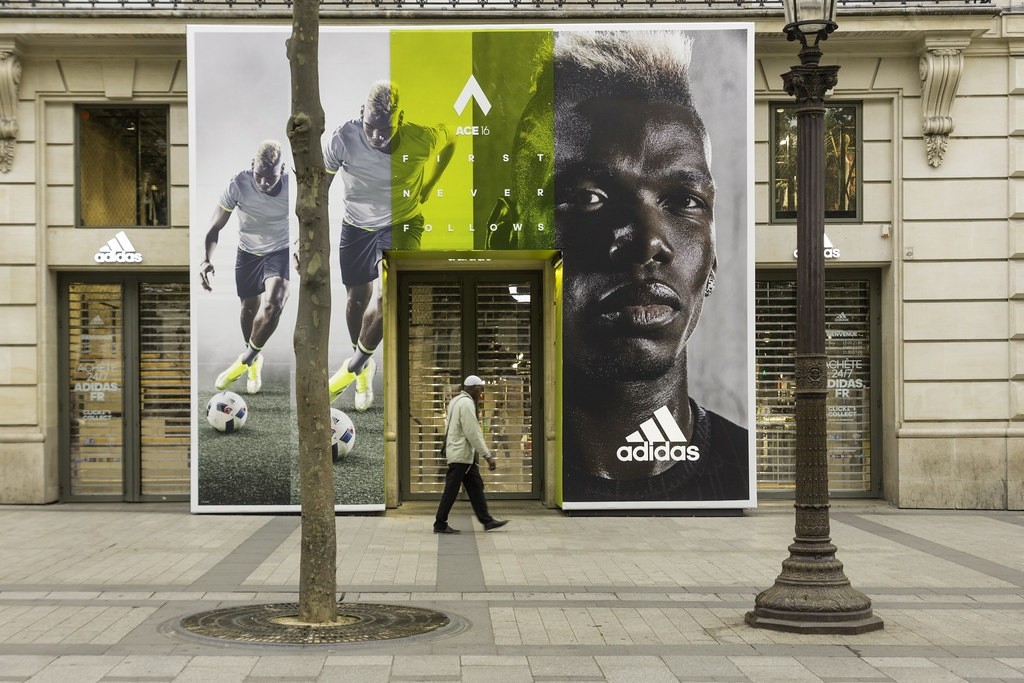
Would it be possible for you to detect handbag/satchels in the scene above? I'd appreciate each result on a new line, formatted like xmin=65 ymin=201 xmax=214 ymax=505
xmin=441 ymin=441 xmax=447 ymax=455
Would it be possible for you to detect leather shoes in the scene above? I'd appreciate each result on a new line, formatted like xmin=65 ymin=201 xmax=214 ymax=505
xmin=434 ymin=525 xmax=461 ymax=534
xmin=485 ymin=519 xmax=509 ymax=530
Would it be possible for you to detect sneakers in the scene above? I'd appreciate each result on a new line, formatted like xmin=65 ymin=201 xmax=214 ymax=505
xmin=247 ymin=353 xmax=264 ymax=394
xmin=215 ymin=354 xmax=250 ymax=390
xmin=354 ymin=357 xmax=377 ymax=412
xmin=329 ymin=358 xmax=358 ymax=405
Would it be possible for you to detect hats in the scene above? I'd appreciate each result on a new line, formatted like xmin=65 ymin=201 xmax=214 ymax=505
xmin=464 ymin=375 xmax=486 ymax=387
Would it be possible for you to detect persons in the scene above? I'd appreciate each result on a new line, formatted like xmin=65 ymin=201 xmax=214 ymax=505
xmin=199 ymin=140 xmax=299 ymax=395
xmin=433 ymin=375 xmax=511 ymax=534
xmin=323 ymin=80 xmax=456 ymax=416
xmin=484 ymin=29 xmax=750 ymax=502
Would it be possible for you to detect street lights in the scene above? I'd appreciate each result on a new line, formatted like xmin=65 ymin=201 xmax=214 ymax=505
xmin=744 ymin=0 xmax=884 ymax=637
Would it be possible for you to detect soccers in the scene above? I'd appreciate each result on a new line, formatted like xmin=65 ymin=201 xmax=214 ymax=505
xmin=206 ymin=390 xmax=249 ymax=434
xmin=330 ymin=407 xmax=356 ymax=463
